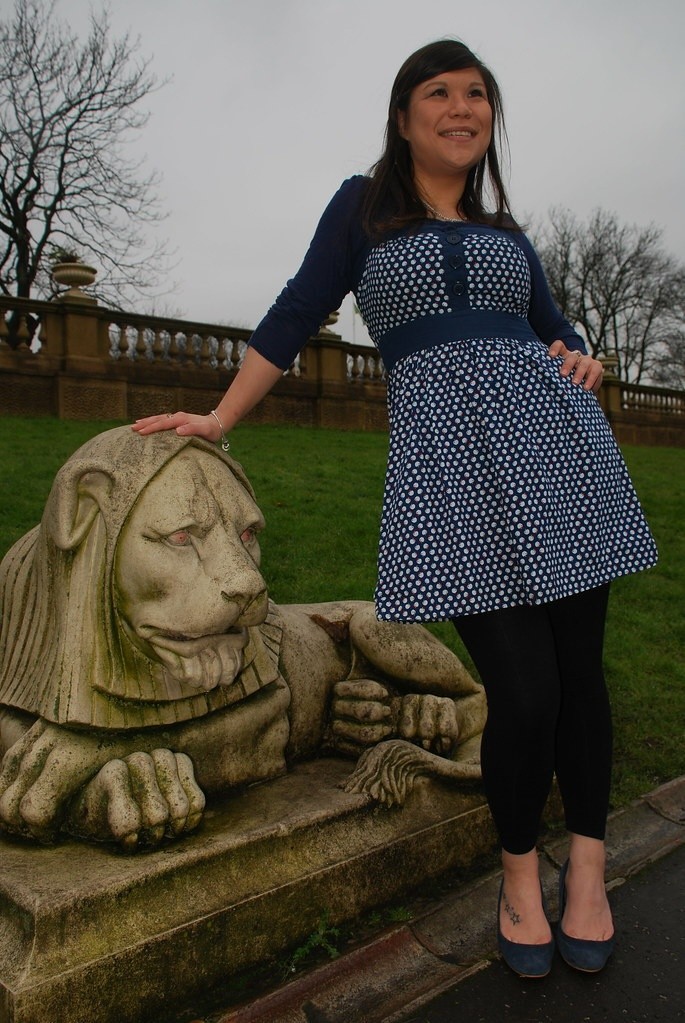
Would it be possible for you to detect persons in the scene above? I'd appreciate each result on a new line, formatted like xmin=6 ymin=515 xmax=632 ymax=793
xmin=131 ymin=39 xmax=658 ymax=977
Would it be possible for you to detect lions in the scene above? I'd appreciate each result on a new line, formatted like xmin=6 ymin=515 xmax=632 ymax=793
xmin=0 ymin=415 xmax=492 ymax=856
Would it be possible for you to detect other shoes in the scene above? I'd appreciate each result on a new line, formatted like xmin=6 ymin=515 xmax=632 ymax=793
xmin=497 ymin=875 xmax=552 ymax=978
xmin=558 ymin=857 xmax=616 ymax=972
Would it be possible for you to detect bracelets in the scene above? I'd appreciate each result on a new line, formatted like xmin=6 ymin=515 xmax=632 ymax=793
xmin=210 ymin=410 xmax=229 ymax=451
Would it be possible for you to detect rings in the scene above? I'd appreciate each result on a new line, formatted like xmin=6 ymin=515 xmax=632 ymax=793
xmin=166 ymin=413 xmax=173 ymax=418
xmin=570 ymin=350 xmax=582 ymax=363
